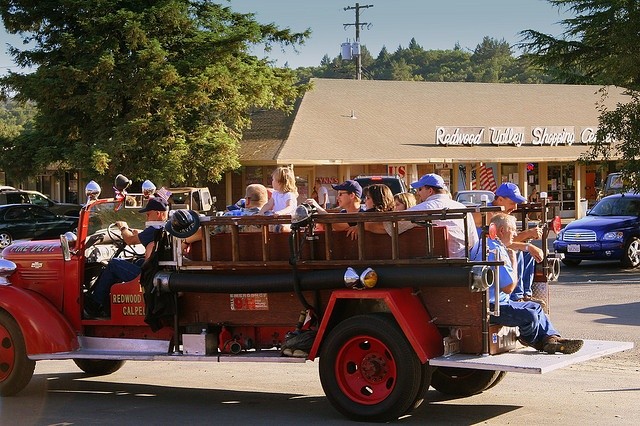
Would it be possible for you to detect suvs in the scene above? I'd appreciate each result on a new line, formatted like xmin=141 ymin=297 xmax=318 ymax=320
xmin=351 ymin=176 xmax=405 ymax=211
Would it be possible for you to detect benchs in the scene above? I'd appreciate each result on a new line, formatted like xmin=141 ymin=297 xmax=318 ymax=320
xmin=534 ymin=266 xmax=547 ymax=283
xmin=207 ymin=225 xmax=449 ymax=258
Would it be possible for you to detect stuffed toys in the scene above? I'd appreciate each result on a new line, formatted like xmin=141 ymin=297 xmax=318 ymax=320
xmin=112 ymin=174 xmax=132 ymax=199
xmin=141 ymin=179 xmax=156 ymax=199
xmin=85 ymin=181 xmax=101 ymax=206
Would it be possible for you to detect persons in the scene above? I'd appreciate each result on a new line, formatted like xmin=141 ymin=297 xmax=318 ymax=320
xmin=315 ymin=180 xmax=330 ymax=210
xmin=255 ymin=167 xmax=300 ymax=232
xmin=469 ymin=213 xmax=584 ymax=355
xmin=313 ymin=223 xmax=332 ymax=235
xmin=304 ymin=184 xmax=396 ymax=231
xmin=331 ymin=180 xmax=367 ymax=232
xmin=345 ymin=174 xmax=479 ymax=258
xmin=528 ymin=188 xmax=542 ymax=220
xmin=394 ymin=192 xmax=416 ymax=211
xmin=84 ymin=199 xmax=168 ymax=310
xmin=471 ymin=182 xmax=546 ymax=308
xmin=181 ymin=183 xmax=294 ymax=251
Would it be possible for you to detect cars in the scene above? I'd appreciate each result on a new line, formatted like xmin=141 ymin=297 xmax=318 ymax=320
xmin=0 ymin=203 xmax=77 ymax=248
xmin=454 ymin=190 xmax=495 ymax=208
xmin=1 ymin=190 xmax=79 ymax=224
xmin=553 ymin=193 xmax=640 ymax=268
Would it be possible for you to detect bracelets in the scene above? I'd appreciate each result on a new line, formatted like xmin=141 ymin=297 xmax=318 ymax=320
xmin=525 ymin=243 xmax=529 ymax=252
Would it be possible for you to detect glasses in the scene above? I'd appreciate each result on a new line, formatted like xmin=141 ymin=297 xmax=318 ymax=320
xmin=339 ymin=192 xmax=348 ymax=196
xmin=417 ymin=188 xmax=427 ymax=192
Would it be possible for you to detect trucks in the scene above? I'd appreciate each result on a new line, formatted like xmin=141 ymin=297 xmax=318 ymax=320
xmin=0 ymin=173 xmax=635 ymax=420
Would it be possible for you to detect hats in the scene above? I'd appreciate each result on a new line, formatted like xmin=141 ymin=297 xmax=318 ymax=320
xmin=411 ymin=174 xmax=444 ymax=188
xmin=331 ymin=180 xmax=362 ymax=198
xmin=138 ymin=197 xmax=168 ymax=213
xmin=496 ymin=183 xmax=526 ymax=203
xmin=165 ymin=210 xmax=200 ymax=238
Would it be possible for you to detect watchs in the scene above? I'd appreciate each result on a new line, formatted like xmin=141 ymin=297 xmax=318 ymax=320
xmin=182 ymin=239 xmax=190 ymax=245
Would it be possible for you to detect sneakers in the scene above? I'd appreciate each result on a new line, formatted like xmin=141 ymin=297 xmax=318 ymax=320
xmin=544 ymin=335 xmax=584 ymax=354
xmin=518 ymin=335 xmax=543 ymax=352
